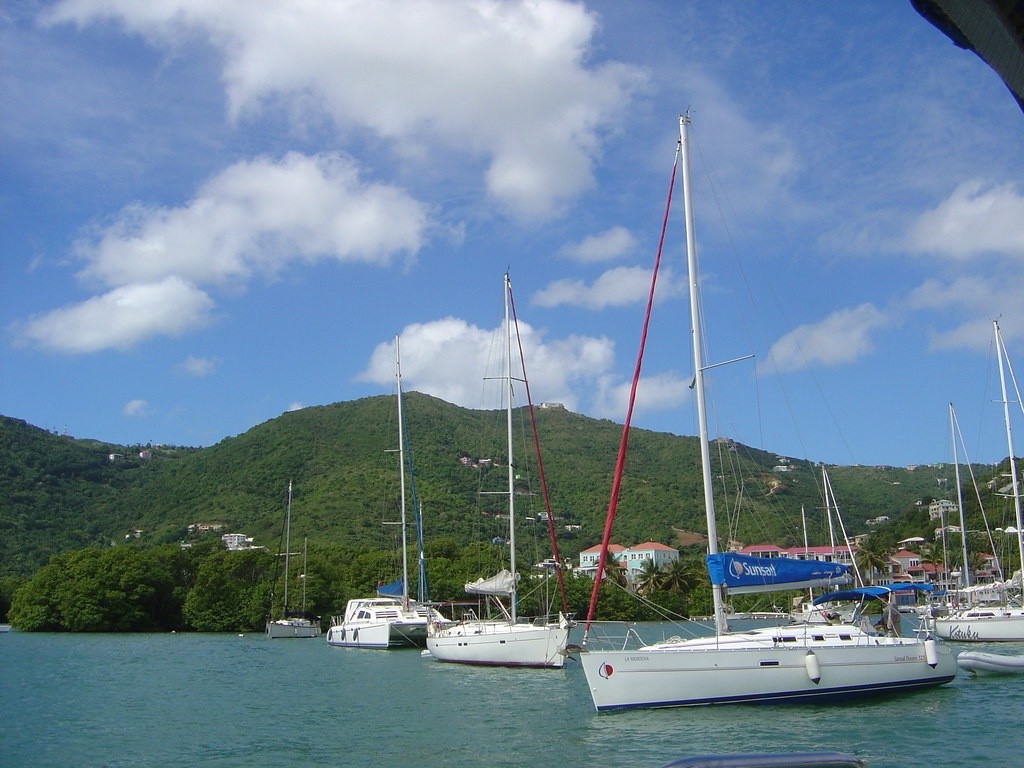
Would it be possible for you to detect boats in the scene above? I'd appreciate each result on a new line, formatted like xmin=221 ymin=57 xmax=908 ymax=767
xmin=957 ymin=652 xmax=1024 ymax=672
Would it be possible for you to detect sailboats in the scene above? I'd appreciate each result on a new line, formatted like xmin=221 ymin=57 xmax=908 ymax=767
xmin=327 ymin=334 xmax=452 ymax=647
xmin=266 ymin=480 xmax=317 ymax=638
xmin=690 ymin=312 xmax=1024 ymax=641
xmin=580 ymin=106 xmax=956 ymax=712
xmin=426 ymin=297 xmax=578 ymax=667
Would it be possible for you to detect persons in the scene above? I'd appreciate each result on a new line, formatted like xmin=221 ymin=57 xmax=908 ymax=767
xmin=828 ymin=611 xmax=842 ymax=625
xmin=881 ymin=594 xmax=902 ymax=638
